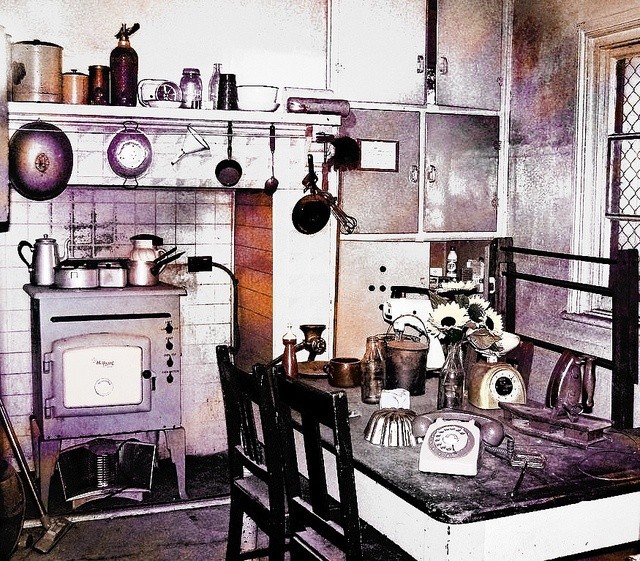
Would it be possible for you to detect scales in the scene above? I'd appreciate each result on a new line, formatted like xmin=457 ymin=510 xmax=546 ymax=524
xmin=467 ymin=329 xmax=527 ymax=409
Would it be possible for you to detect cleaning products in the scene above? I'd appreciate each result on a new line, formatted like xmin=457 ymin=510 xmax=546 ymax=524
xmin=447 ymin=246 xmax=457 ymax=276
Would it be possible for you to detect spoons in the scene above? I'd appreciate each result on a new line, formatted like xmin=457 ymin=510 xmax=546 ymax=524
xmin=265 ymin=125 xmax=279 ymax=196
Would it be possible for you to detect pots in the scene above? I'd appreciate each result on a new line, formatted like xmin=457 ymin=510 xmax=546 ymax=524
xmin=63 ymin=69 xmax=89 ymax=105
xmin=12 ymin=39 xmax=63 ymax=104
xmin=215 ymin=121 xmax=242 ymax=186
xmin=55 ymin=260 xmax=98 ymax=289
xmin=98 ymin=264 xmax=127 ymax=288
xmin=291 ymin=154 xmax=330 ymax=235
xmin=324 ymin=357 xmax=361 ymax=388
xmin=7 ymin=117 xmax=74 ymax=202
xmin=128 ymin=247 xmax=186 ymax=286
xmin=106 ymin=120 xmax=153 ymax=189
xmin=236 ymin=85 xmax=280 ymax=112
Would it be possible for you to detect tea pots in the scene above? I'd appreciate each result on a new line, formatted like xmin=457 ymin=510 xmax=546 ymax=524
xmin=17 ymin=233 xmax=71 ymax=286
xmin=129 ymin=234 xmax=168 ymax=274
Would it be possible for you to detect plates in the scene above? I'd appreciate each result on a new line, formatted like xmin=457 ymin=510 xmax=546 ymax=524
xmin=148 ymin=100 xmax=182 ymax=108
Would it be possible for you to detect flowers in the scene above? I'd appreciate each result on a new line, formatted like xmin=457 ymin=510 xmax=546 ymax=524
xmin=424 ymin=293 xmax=506 ymax=368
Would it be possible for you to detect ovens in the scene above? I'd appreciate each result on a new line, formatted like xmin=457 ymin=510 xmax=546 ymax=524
xmin=23 ymin=281 xmax=189 ymax=513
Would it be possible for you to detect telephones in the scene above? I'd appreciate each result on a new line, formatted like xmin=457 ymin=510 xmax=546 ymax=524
xmin=412 ymin=408 xmax=515 ymax=476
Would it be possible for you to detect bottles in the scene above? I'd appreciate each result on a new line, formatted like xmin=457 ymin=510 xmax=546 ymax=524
xmin=282 ymin=322 xmax=300 ymax=381
xmin=360 ymin=336 xmax=387 ymax=404
xmin=219 ymin=73 xmax=238 ymax=110
xmin=209 ymin=63 xmax=223 ymax=110
xmin=446 ymin=246 xmax=458 ymax=283
xmin=179 ymin=69 xmax=203 ymax=109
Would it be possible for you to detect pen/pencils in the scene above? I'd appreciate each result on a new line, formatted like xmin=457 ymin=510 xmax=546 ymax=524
xmin=510 ymin=462 xmax=528 ymax=497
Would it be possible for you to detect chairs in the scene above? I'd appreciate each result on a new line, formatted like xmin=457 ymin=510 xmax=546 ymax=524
xmin=213 ymin=344 xmax=318 ymax=560
xmin=272 ymin=364 xmax=408 ymax=558
xmin=478 ymin=337 xmax=534 ymax=397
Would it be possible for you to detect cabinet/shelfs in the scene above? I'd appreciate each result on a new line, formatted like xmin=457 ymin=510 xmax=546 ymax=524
xmin=338 ymin=240 xmax=501 ymax=364
xmin=338 ymin=106 xmax=502 ymax=233
xmin=326 ymin=1 xmax=502 ymax=110
